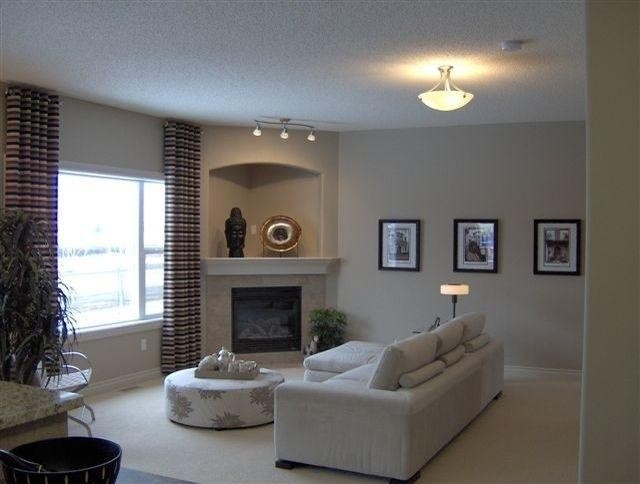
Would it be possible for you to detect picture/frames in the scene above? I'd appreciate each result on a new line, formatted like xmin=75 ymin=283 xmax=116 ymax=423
xmin=533 ymin=219 xmax=580 ymax=276
xmin=378 ymin=219 xmax=419 ymax=271
xmin=453 ymin=219 xmax=498 ymax=273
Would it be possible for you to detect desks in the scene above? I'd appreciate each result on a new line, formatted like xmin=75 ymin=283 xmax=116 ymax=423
xmin=0 ymin=381 xmax=84 ymax=480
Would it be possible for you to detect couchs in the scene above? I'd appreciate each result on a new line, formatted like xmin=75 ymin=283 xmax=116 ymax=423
xmin=274 ymin=314 xmax=504 ymax=483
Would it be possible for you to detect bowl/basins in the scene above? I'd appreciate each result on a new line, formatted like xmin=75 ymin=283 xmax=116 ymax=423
xmin=4 ymin=438 xmax=122 ymax=484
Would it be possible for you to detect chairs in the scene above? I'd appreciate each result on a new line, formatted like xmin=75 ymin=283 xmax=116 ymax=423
xmin=27 ymin=350 xmax=96 ymax=437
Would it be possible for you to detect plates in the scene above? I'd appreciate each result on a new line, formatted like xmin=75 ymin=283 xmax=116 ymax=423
xmin=260 ymin=216 xmax=301 ymax=252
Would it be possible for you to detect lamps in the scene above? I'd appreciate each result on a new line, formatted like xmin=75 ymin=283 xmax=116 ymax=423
xmin=418 ymin=67 xmax=474 ymax=112
xmin=252 ymin=118 xmax=316 ymax=142
xmin=440 ymin=283 xmax=469 ymax=319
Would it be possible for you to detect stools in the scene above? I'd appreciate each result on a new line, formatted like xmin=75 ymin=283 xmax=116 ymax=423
xmin=164 ymin=362 xmax=285 ymax=429
